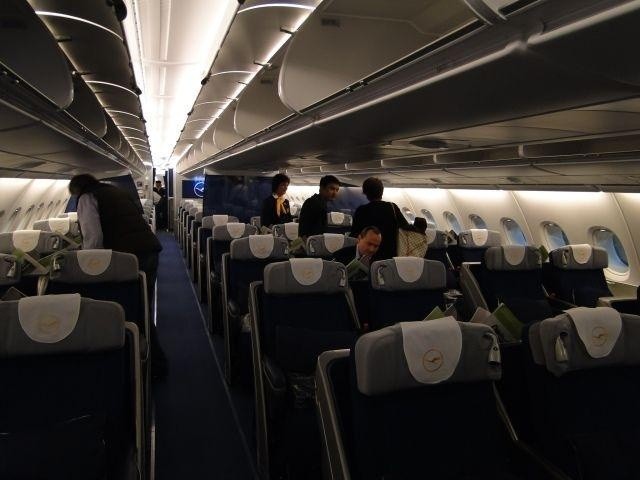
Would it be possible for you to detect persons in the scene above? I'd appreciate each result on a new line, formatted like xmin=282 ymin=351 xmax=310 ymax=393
xmin=349 ymin=177 xmax=426 ymax=257
xmin=67 ymin=173 xmax=172 ymax=388
xmin=330 ymin=226 xmax=392 ymax=266
xmin=298 ymin=176 xmax=340 ymax=237
xmin=154 ymin=180 xmax=167 ymax=230
xmin=261 ymin=173 xmax=293 ymax=224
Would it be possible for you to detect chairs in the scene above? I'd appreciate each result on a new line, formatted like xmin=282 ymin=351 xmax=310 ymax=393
xmin=177 ymin=195 xmax=640 ymax=473
xmin=0 ymin=195 xmax=160 ymax=480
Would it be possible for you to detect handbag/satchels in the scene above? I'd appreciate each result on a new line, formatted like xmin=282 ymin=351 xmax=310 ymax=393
xmin=397 ymin=230 xmax=428 ymax=260
